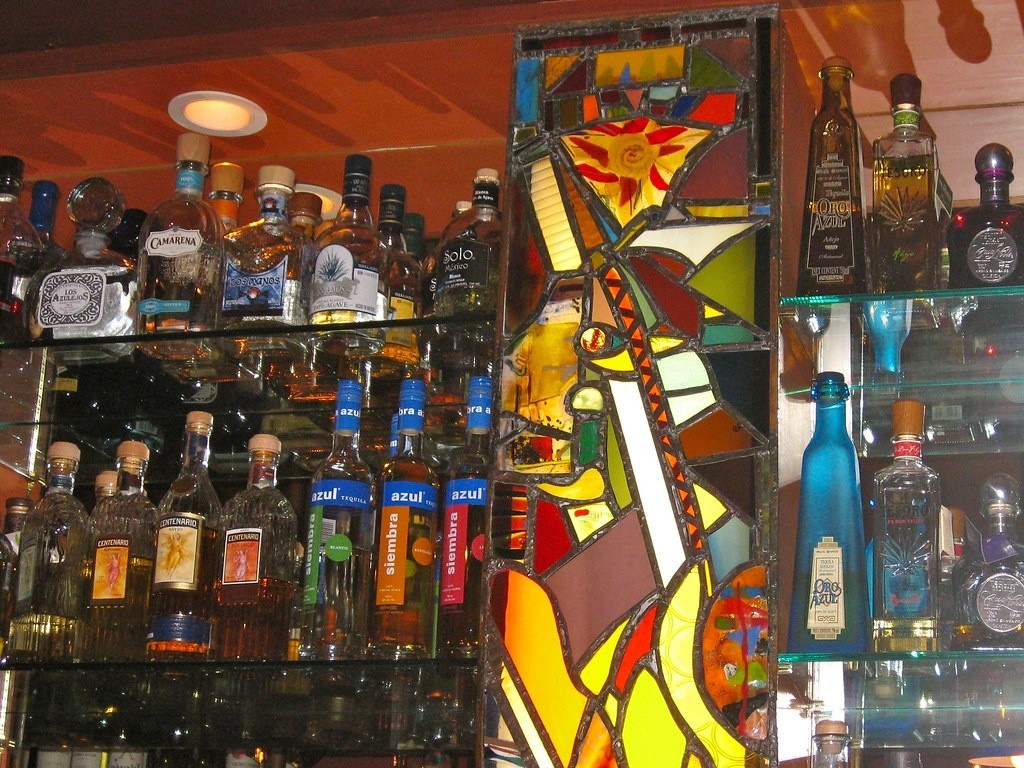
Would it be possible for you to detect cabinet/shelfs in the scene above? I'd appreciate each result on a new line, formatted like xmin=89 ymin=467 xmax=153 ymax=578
xmin=0 ymin=309 xmax=500 ymax=768
xmin=779 ymin=286 xmax=1024 ymax=768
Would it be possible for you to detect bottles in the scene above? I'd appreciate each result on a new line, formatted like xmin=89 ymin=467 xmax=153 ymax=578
xmin=812 ymin=719 xmax=852 ymax=768
xmin=797 ymin=57 xmax=868 ymax=333
xmin=0 ymin=134 xmax=490 ymax=671
xmin=788 ymin=372 xmax=868 ymax=655
xmin=951 ymin=475 xmax=1024 ymax=648
xmin=0 ymin=674 xmax=475 ymax=768
xmin=946 ymin=142 xmax=1024 ymax=330
xmin=872 ymin=399 xmax=938 ymax=677
xmin=871 ymin=74 xmax=943 ymax=333
